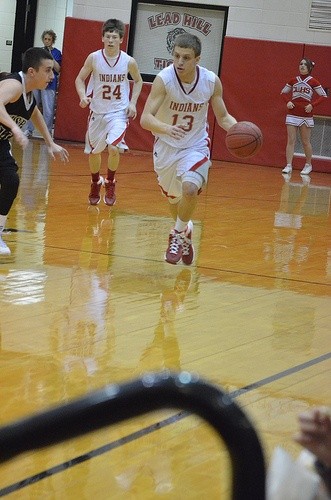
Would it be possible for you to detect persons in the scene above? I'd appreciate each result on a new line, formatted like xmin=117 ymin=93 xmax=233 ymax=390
xmin=75 ymin=19 xmax=143 ymax=206
xmin=140 ymin=33 xmax=238 ymax=265
xmin=21 ymin=31 xmax=62 ymax=139
xmin=135 ymin=269 xmax=194 ymax=370
xmin=0 ymin=47 xmax=70 ymax=257
xmin=19 ymin=141 xmax=49 ymax=228
xmin=48 ymin=206 xmax=114 ymax=392
xmin=279 ymin=57 xmax=328 ymax=175
xmin=292 ymin=409 xmax=331 ymax=500
xmin=265 ymin=175 xmax=311 ymax=289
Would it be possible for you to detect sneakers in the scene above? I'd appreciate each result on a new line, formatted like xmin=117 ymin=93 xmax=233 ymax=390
xmin=282 ymin=165 xmax=292 ymax=173
xmin=104 ymin=177 xmax=116 ymax=206
xmin=300 ymin=164 xmax=312 ymax=174
xmin=182 ymin=221 xmax=195 ymax=265
xmin=88 ymin=176 xmax=103 ymax=204
xmin=165 ymin=229 xmax=186 ymax=264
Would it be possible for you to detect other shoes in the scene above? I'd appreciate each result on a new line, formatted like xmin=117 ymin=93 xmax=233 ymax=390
xmin=0 ymin=238 xmax=10 ymax=254
xmin=24 ymin=130 xmax=32 ymax=136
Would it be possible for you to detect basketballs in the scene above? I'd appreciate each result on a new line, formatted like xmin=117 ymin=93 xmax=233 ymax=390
xmin=226 ymin=121 xmax=263 ymax=160
